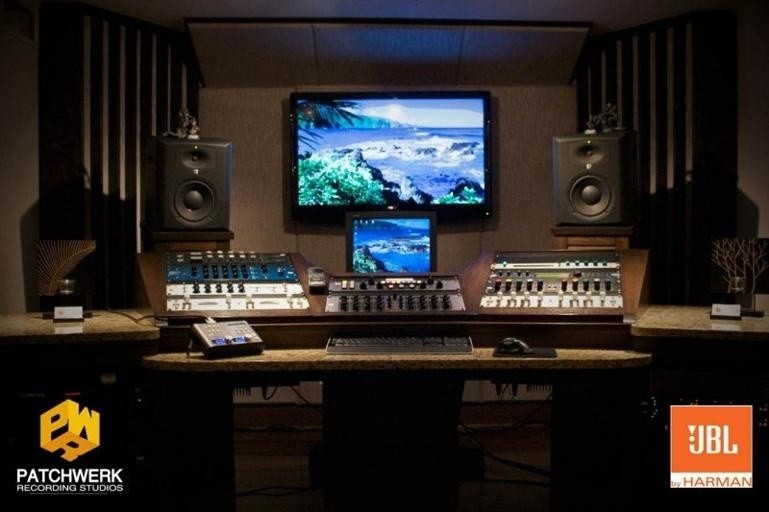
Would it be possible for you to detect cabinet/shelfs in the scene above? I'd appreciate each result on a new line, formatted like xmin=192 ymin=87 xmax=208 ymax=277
xmin=1 ymin=308 xmax=164 ymax=511
xmin=628 ymin=304 xmax=769 ymax=511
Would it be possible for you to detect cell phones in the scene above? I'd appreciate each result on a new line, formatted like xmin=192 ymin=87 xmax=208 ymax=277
xmin=307 ymin=266 xmax=326 ymax=287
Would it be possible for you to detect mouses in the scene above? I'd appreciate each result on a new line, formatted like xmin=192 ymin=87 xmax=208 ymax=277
xmin=496 ymin=336 xmax=531 ymax=356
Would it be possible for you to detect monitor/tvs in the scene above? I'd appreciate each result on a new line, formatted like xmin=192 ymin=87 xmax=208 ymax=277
xmin=288 ymin=90 xmax=494 ymax=223
xmin=344 ymin=211 xmax=438 ymax=272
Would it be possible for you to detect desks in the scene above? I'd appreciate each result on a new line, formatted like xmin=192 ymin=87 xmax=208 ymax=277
xmin=137 ymin=225 xmax=654 ymax=510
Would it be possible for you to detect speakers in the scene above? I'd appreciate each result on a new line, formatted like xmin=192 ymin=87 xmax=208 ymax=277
xmin=552 ymin=132 xmax=628 ymax=226
xmin=150 ymin=138 xmax=232 ymax=231
